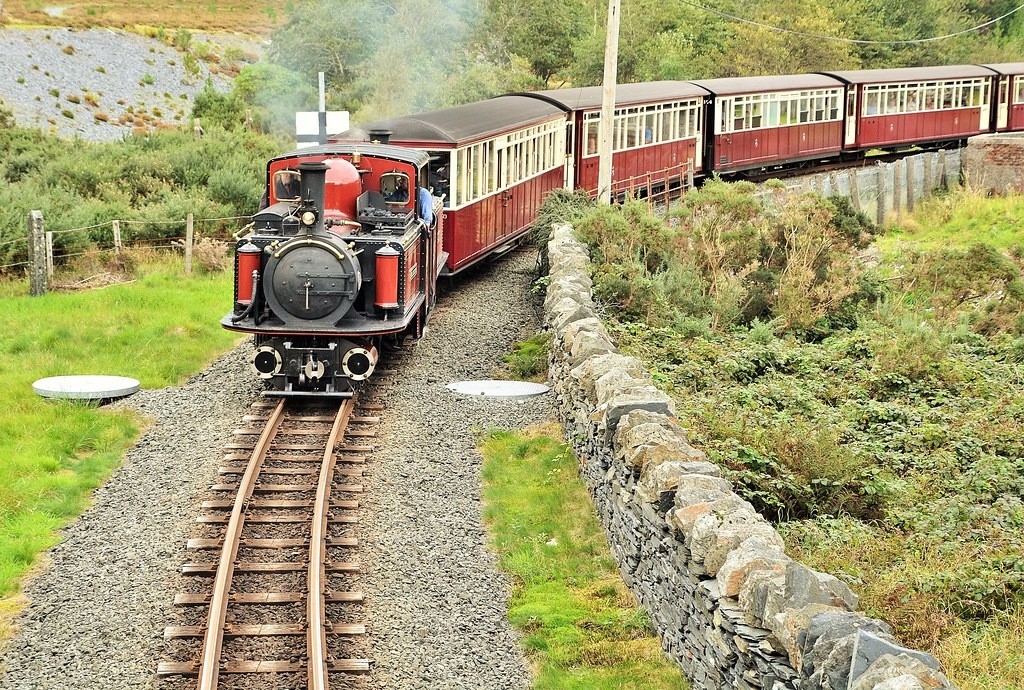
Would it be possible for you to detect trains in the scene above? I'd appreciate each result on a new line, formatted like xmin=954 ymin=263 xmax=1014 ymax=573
xmin=224 ymin=63 xmax=1024 ymax=396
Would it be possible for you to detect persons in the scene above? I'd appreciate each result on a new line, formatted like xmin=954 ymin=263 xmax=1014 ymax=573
xmin=639 ymin=124 xmax=651 ymax=144
xmin=388 ymin=172 xmax=432 ymax=229
xmin=868 ymin=92 xmax=968 ymax=114
xmin=259 ymin=172 xmax=301 ymax=211
